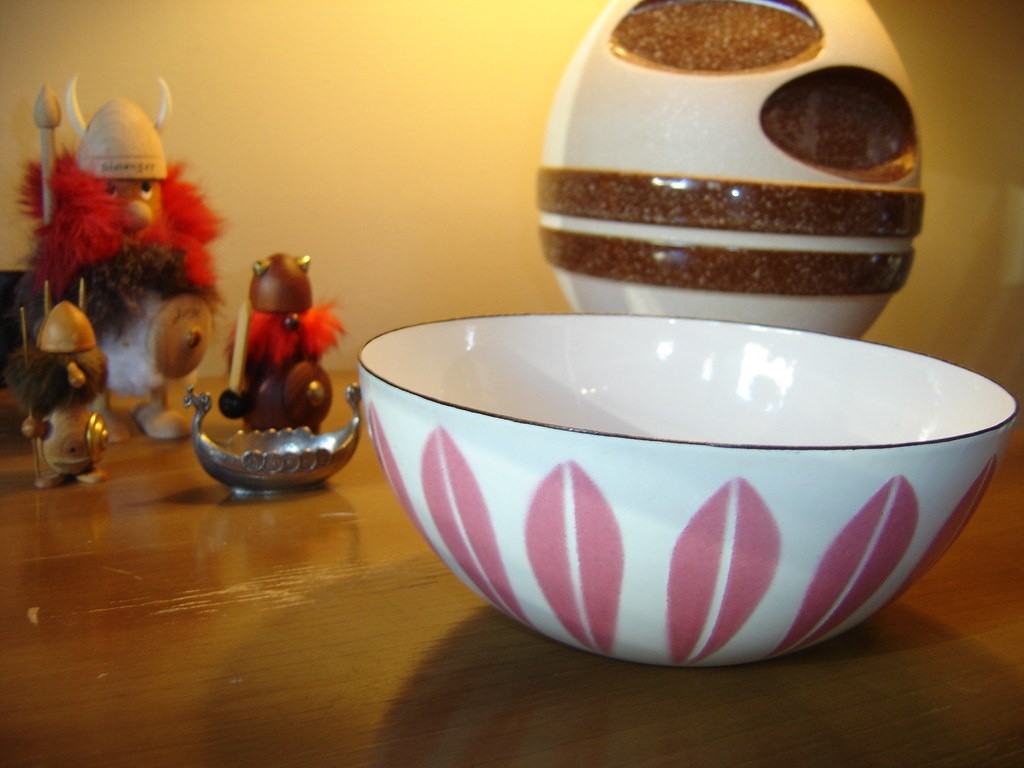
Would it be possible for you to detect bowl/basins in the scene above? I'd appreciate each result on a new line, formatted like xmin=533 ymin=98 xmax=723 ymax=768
xmin=357 ymin=312 xmax=1018 ymax=671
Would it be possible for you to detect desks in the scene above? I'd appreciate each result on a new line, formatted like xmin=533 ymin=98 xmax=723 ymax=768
xmin=0 ymin=368 xmax=1024 ymax=768
